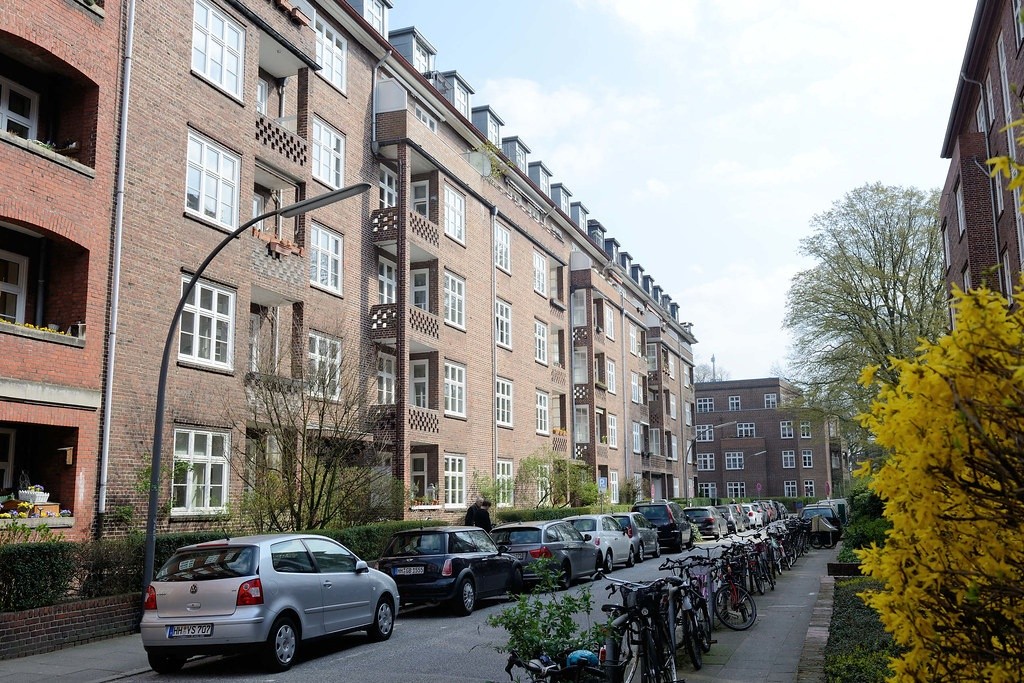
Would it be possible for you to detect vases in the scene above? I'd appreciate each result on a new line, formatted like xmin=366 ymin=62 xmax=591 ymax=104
xmin=19 ymin=490 xmax=50 ymax=503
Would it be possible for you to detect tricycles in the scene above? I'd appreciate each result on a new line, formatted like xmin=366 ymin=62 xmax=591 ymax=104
xmin=502 ymin=569 xmax=686 ymax=683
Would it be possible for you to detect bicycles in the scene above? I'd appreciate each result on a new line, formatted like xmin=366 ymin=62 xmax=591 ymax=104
xmin=597 ymin=499 xmax=847 ymax=683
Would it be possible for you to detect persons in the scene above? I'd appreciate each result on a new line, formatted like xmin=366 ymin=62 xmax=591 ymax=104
xmin=480 ymin=500 xmax=492 ymax=533
xmin=465 ymin=497 xmax=484 ymax=528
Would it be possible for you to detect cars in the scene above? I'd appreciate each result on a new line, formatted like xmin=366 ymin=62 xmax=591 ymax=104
xmin=487 ymin=519 xmax=603 ymax=590
xmin=563 ymin=514 xmax=636 ymax=573
xmin=374 ymin=524 xmax=525 ymax=616
xmin=140 ymin=532 xmax=402 ymax=672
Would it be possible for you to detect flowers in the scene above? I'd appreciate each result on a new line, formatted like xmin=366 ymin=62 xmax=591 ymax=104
xmin=0 ymin=502 xmax=72 ymax=518
xmin=28 ymin=484 xmax=44 ymax=492
xmin=0 ymin=318 xmax=72 ymax=336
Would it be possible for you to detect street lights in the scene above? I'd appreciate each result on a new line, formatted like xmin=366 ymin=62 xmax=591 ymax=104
xmin=683 ymin=419 xmax=767 ymax=499
xmin=138 ymin=180 xmax=377 ymax=610
